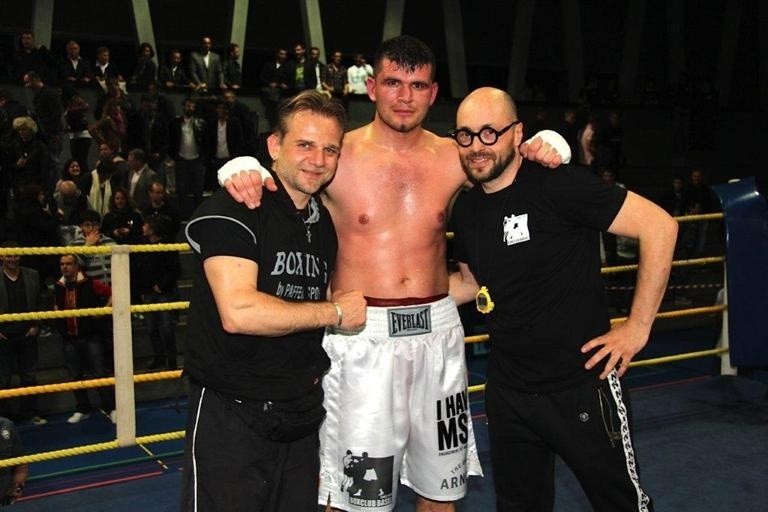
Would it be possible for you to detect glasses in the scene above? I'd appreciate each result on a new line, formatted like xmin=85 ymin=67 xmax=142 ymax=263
xmin=447 ymin=121 xmax=519 ymax=147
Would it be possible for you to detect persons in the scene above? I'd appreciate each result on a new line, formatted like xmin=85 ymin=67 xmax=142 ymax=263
xmin=0 ymin=30 xmax=374 ymax=424
xmin=217 ymin=35 xmax=572 ymax=512
xmin=181 ymin=89 xmax=367 ymax=512
xmin=0 ymin=416 xmax=28 ymax=512
xmin=523 ymin=105 xmax=712 ymax=315
xmin=445 ymin=86 xmax=680 ymax=512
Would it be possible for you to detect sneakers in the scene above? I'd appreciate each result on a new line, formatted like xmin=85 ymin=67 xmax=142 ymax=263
xmin=31 ymin=417 xmax=47 ymax=426
xmin=67 ymin=412 xmax=91 ymax=424
xmin=106 ymin=410 xmax=117 ymax=424
